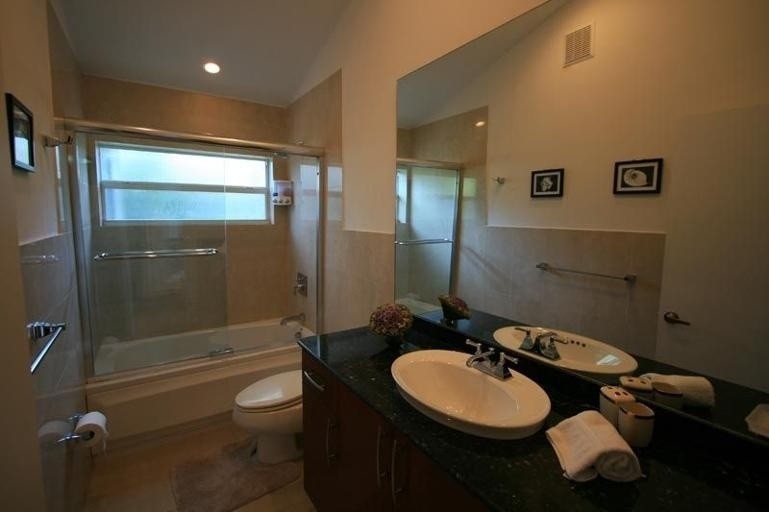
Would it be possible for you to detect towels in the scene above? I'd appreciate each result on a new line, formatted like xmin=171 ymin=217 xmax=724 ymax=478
xmin=636 ymin=368 xmax=716 ymax=410
xmin=542 ymin=409 xmax=645 ymax=489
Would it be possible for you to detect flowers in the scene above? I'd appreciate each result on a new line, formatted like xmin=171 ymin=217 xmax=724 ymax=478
xmin=435 ymin=291 xmax=473 ymax=325
xmin=367 ymin=301 xmax=412 ymax=343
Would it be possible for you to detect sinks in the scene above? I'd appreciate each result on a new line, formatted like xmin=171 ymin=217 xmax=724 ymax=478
xmin=389 ymin=349 xmax=552 ymax=440
xmin=493 ymin=325 xmax=639 ymax=374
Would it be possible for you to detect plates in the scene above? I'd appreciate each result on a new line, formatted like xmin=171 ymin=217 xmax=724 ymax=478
xmin=746 ymin=402 xmax=769 ymax=437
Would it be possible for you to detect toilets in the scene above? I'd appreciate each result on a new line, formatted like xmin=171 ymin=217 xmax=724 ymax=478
xmin=232 ymin=370 xmax=304 ymax=467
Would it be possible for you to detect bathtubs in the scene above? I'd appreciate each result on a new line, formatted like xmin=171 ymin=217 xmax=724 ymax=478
xmin=92 ymin=315 xmax=316 ymax=377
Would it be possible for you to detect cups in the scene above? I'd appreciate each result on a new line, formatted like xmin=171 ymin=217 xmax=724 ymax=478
xmin=651 ymin=380 xmax=683 ymax=409
xmin=617 ymin=402 xmax=655 ymax=445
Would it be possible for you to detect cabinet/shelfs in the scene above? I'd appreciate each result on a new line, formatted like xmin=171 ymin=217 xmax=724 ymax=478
xmin=336 ymin=378 xmax=498 ymax=512
xmin=296 ymin=345 xmax=340 ymax=512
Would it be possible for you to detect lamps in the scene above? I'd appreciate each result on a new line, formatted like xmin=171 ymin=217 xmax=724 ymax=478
xmin=41 ymin=133 xmax=74 ymax=148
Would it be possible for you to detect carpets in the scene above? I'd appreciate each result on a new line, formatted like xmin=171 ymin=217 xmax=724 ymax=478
xmin=166 ymin=423 xmax=303 ymax=511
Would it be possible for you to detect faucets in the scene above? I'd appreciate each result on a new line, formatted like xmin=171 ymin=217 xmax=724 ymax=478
xmin=532 ymin=331 xmax=568 ymax=355
xmin=279 ymin=312 xmax=305 ymax=327
xmin=465 ymin=347 xmax=496 ymax=369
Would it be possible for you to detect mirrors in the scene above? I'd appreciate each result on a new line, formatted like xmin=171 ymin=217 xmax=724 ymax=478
xmin=391 ymin=4 xmax=768 ymax=398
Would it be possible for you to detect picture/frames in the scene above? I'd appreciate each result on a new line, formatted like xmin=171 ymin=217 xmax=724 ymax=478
xmin=4 ymin=90 xmax=39 ymax=177
xmin=528 ymin=165 xmax=565 ymax=200
xmin=607 ymin=156 xmax=664 ymax=195
